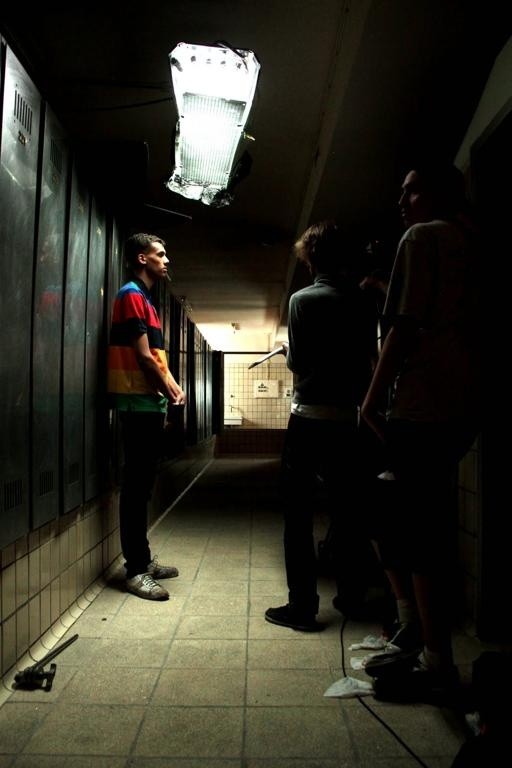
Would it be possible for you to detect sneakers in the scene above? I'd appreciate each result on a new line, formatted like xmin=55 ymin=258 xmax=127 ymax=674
xmin=127 ymin=573 xmax=169 ymax=600
xmin=145 ymin=561 xmax=178 ymax=578
xmin=364 ymin=624 xmax=461 ymax=703
xmin=266 ymin=606 xmax=317 ymax=630
xmin=334 ymin=595 xmax=371 ymax=623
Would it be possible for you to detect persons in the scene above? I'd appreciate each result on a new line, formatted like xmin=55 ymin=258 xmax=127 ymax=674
xmin=264 ymin=156 xmax=490 ymax=700
xmin=105 ymin=233 xmax=187 ymax=602
